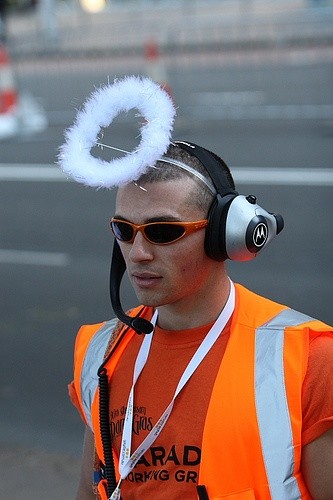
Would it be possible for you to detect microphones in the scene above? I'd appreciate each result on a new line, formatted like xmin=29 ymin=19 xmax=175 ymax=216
xmin=110 ymin=237 xmax=156 ymax=334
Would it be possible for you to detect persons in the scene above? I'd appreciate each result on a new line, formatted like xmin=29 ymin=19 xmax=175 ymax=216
xmin=67 ymin=142 xmax=333 ymax=500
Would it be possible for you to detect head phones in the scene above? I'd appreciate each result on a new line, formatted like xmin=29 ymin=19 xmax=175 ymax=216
xmin=168 ymin=140 xmax=284 ymax=262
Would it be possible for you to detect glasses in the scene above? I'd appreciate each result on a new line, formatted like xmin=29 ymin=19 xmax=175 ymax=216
xmin=107 ymin=215 xmax=206 ymax=250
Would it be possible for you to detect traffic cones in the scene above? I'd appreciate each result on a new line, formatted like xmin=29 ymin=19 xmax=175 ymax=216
xmin=140 ymin=40 xmax=170 ymax=98
xmin=0 ymin=43 xmax=25 ymax=139
